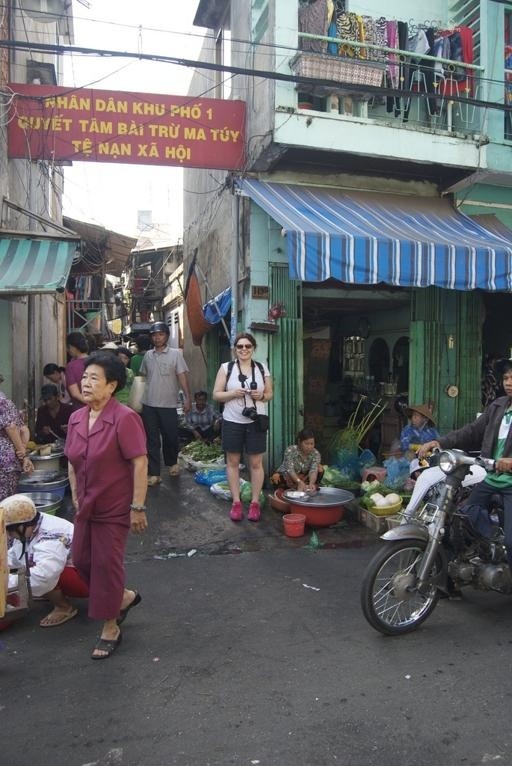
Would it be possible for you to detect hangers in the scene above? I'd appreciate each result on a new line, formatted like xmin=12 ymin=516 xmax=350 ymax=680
xmin=336 ymin=5 xmax=442 ymax=34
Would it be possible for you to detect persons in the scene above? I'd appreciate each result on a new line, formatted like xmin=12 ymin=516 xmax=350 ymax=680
xmin=1 ymin=324 xmax=223 ymax=659
xmin=409 ymin=458 xmax=448 ymax=508
xmin=212 ymin=333 xmax=273 ymax=522
xmin=399 ymin=405 xmax=442 ymax=461
xmin=271 ymin=429 xmax=324 ymax=497
xmin=416 ymin=357 xmax=512 ymax=568
xmin=481 ymin=353 xmax=507 ymax=407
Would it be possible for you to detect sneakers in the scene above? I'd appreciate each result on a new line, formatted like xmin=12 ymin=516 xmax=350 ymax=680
xmin=248 ymin=503 xmax=260 ymax=521
xmin=230 ymin=502 xmax=243 ymax=521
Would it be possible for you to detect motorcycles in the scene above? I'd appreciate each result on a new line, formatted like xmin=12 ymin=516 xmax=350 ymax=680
xmin=360 ymin=435 xmax=511 ymax=638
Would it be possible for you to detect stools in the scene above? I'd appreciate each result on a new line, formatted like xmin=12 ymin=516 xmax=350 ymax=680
xmin=361 ymin=466 xmax=387 ymax=482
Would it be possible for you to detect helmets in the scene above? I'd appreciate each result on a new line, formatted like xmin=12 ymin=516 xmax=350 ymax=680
xmin=149 ymin=321 xmax=170 ymax=336
xmin=0 ymin=494 xmax=37 ymax=526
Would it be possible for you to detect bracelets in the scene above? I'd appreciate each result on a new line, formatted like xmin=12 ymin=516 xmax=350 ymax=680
xmin=293 ymin=478 xmax=301 ymax=485
xmin=260 ymin=394 xmax=265 ymax=401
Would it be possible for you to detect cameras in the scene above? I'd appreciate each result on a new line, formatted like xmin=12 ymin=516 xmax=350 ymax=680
xmin=242 ymin=406 xmax=258 ymax=423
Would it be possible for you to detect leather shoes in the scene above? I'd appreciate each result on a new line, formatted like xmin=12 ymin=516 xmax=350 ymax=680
xmin=439 ymin=586 xmax=462 ymax=600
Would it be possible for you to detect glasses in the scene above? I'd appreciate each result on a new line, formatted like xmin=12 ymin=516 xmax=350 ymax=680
xmin=237 ymin=345 xmax=253 ymax=349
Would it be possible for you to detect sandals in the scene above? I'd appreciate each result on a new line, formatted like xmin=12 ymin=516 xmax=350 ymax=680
xmin=169 ymin=464 xmax=179 ymax=476
xmin=147 ymin=476 xmax=162 ymax=486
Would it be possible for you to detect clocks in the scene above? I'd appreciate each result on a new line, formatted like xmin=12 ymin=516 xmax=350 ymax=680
xmin=358 ymin=317 xmax=370 ymax=337
xmin=447 ymin=385 xmax=459 ymax=398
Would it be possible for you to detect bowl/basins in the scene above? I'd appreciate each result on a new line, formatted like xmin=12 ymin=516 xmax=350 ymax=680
xmin=17 ymin=469 xmax=69 ymax=497
xmin=0 ymin=492 xmax=64 ymax=516
xmin=290 ymin=504 xmax=343 ymax=527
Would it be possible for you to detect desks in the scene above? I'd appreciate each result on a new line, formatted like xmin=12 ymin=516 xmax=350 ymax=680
xmin=363 ymin=395 xmax=408 ymax=461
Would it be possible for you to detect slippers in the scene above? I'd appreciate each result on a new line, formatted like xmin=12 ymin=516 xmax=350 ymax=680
xmin=40 ymin=606 xmax=78 ymax=627
xmin=117 ymin=590 xmax=142 ymax=625
xmin=91 ymin=626 xmax=122 ymax=659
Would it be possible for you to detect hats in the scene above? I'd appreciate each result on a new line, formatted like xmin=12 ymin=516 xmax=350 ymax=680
xmin=100 ymin=342 xmax=119 ymax=350
xmin=404 ymin=406 xmax=436 ymax=428
xmin=492 ymin=347 xmax=512 ymax=370
xmin=39 ymin=384 xmax=57 ymax=400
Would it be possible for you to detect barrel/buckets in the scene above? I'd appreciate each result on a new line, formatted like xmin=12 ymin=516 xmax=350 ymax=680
xmin=283 ymin=514 xmax=306 ymax=538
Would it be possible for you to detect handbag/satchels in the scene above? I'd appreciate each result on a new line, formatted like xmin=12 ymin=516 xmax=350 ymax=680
xmin=255 ymin=415 xmax=269 ymax=432
xmin=127 ymin=376 xmax=145 ymax=414
